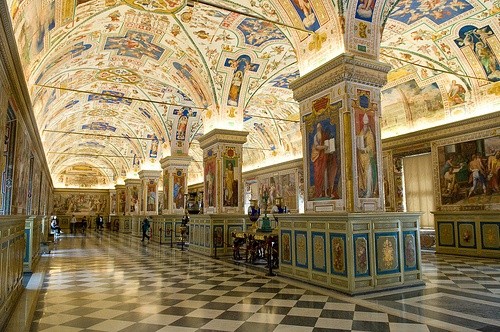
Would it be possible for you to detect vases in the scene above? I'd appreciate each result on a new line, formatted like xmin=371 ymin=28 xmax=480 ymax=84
xmin=186 ymin=191 xmax=200 ymax=213
xmin=247 ymin=200 xmax=260 ymax=221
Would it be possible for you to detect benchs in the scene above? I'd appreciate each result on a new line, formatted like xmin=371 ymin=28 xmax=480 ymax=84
xmin=41 ymin=241 xmax=51 ymax=254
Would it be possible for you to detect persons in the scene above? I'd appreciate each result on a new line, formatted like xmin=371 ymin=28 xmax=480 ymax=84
xmin=69 ymin=215 xmax=77 ymax=234
xmin=141 ymin=216 xmax=151 ymax=241
xmin=100 ymin=216 xmax=104 ymax=228
xmin=81 ymin=216 xmax=87 ymax=233
xmin=51 ymin=220 xmax=64 ymax=236
xmin=96 ymin=214 xmax=101 ymax=233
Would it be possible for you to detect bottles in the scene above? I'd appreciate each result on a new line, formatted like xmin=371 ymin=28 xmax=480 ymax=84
xmin=271 ymin=196 xmax=286 ymax=222
xmin=186 ymin=192 xmax=201 ymax=214
xmin=248 ymin=200 xmax=261 ymax=229
